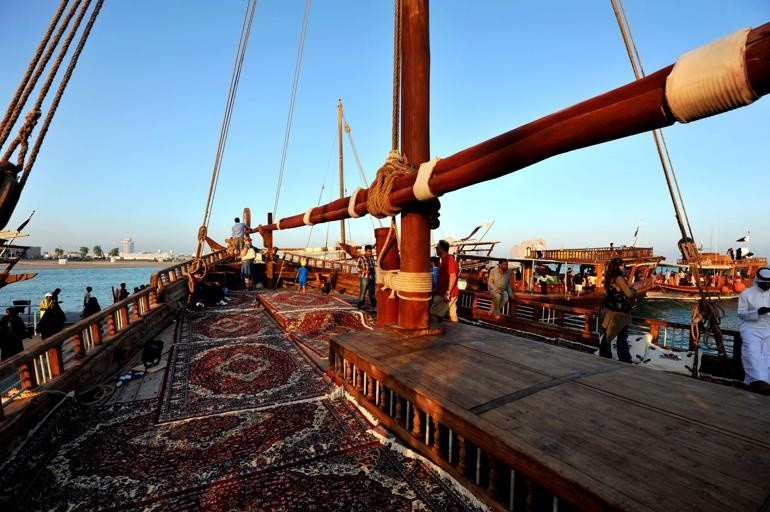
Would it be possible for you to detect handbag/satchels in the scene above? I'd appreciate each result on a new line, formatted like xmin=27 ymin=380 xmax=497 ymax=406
xmin=367 ymin=267 xmax=375 ymax=276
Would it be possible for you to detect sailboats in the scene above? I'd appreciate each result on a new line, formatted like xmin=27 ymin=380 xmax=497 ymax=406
xmin=0 ymin=0 xmax=770 ymax=511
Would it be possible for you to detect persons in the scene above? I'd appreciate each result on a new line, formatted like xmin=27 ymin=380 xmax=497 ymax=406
xmin=238 ymin=237 xmax=256 ymax=291
xmin=356 ymin=245 xmax=377 ymax=310
xmin=429 ymin=237 xmax=654 ymax=365
xmin=232 ymin=217 xmax=252 ymax=247
xmin=193 ymin=270 xmax=233 ymax=305
xmin=294 ymin=259 xmax=309 ymax=295
xmin=1 ymin=283 xmax=149 ymax=358
xmin=737 ymin=268 xmax=769 ymax=391
xmin=654 ymin=266 xmax=756 ymax=292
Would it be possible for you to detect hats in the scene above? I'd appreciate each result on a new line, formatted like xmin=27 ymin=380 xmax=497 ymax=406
xmin=44 ymin=292 xmax=53 ymax=297
